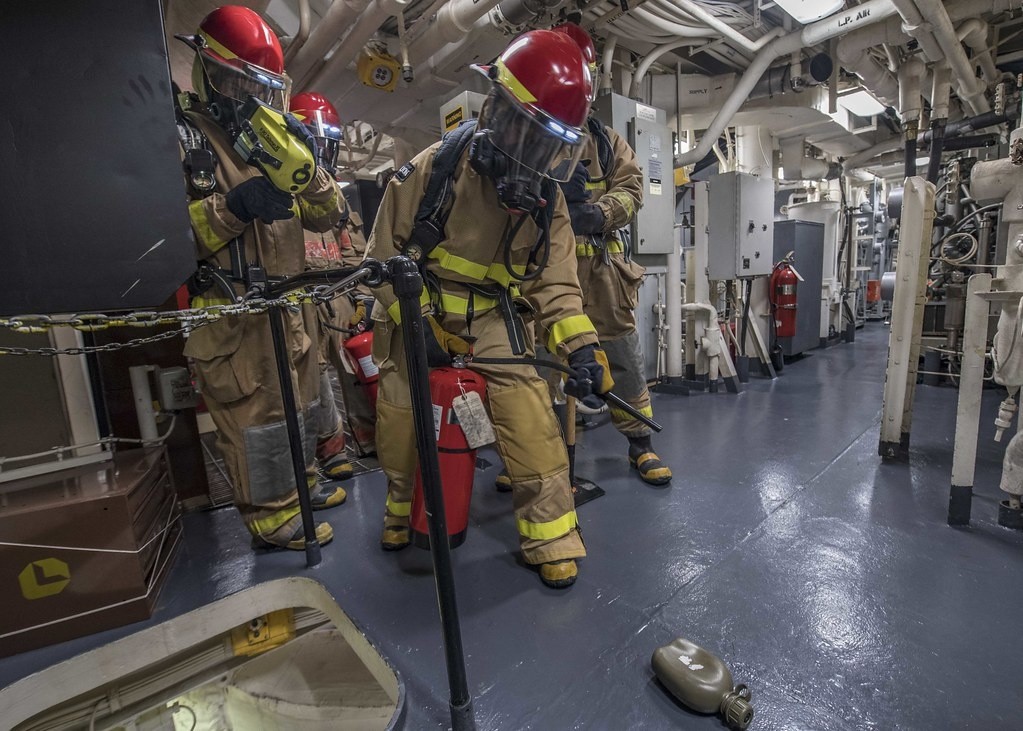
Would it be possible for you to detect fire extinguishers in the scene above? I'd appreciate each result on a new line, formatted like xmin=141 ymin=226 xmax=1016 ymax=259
xmin=323 ymin=320 xmax=380 ymax=412
xmin=769 ymin=250 xmax=805 ymax=343
xmin=407 ymin=333 xmax=664 ymax=551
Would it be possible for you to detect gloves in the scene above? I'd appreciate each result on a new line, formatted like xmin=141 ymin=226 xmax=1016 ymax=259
xmin=563 ymin=345 xmax=605 ymax=409
xmin=421 ymin=312 xmax=470 ymax=367
xmin=569 ymin=201 xmax=604 ymax=234
xmin=350 ymin=298 xmax=375 ymax=332
xmin=548 ymin=157 xmax=592 ymax=196
xmin=224 ymin=175 xmax=295 ymax=224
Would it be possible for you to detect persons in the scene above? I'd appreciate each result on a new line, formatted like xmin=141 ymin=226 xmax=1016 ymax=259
xmin=290 ymin=95 xmax=378 ymax=482
xmin=364 ymin=29 xmax=614 ymax=585
xmin=493 ymin=22 xmax=674 ymax=489
xmin=179 ymin=4 xmax=352 ymax=548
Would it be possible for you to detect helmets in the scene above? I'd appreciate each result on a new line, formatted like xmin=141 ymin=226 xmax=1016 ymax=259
xmin=469 ymin=28 xmax=592 ymax=128
xmin=290 ymin=93 xmax=343 ymax=134
xmin=196 ymin=4 xmax=285 ymax=75
xmin=550 ymin=21 xmax=597 ymax=72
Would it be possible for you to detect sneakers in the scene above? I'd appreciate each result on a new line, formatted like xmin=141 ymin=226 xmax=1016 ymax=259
xmin=494 ymin=467 xmax=513 ymax=491
xmin=323 ymin=459 xmax=354 ymax=478
xmin=381 ymin=508 xmax=411 ymax=549
xmin=539 ymin=559 xmax=578 ymax=587
xmin=285 ymin=520 xmax=334 ymax=549
xmin=311 ymin=486 xmax=347 ymax=509
xmin=627 ymin=441 xmax=672 ymax=485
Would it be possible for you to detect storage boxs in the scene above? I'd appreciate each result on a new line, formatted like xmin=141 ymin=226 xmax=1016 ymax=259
xmin=1 ymin=445 xmax=187 ymax=653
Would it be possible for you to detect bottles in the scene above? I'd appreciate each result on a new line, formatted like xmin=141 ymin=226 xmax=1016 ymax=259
xmin=651 ymin=638 xmax=754 ymax=730
